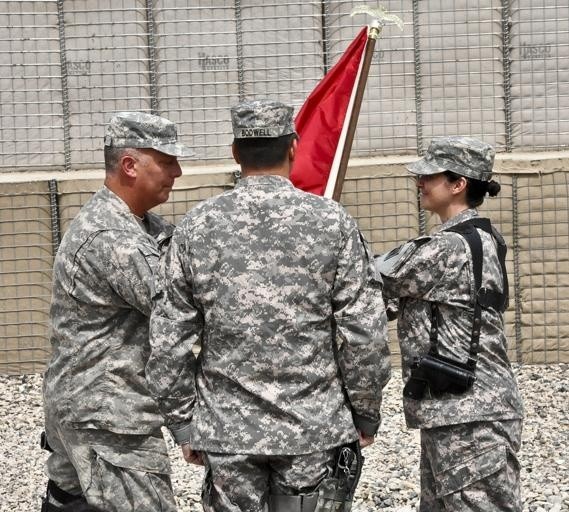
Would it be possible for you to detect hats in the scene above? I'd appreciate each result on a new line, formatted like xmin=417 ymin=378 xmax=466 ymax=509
xmin=103 ymin=111 xmax=197 ymax=158
xmin=231 ymin=99 xmax=300 ymax=140
xmin=404 ymin=137 xmax=496 ymax=182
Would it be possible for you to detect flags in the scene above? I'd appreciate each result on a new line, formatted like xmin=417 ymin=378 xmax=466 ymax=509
xmin=286 ymin=25 xmax=372 ymax=200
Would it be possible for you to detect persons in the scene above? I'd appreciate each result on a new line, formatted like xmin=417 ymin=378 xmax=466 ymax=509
xmin=144 ymin=98 xmax=392 ymax=511
xmin=40 ymin=108 xmax=198 ymax=512
xmin=375 ymin=135 xmax=526 ymax=512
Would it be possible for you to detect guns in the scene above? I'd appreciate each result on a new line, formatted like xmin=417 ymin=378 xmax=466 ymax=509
xmin=403 ymin=356 xmax=474 ymax=401
